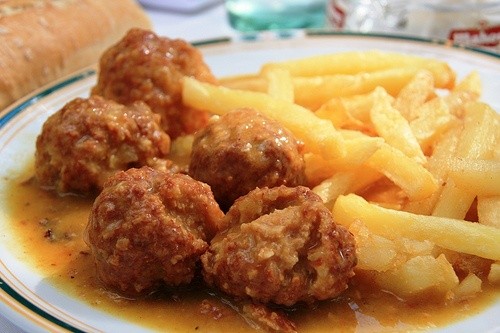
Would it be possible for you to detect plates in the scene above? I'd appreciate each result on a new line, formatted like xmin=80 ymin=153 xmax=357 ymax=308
xmin=0 ymin=30 xmax=500 ymax=332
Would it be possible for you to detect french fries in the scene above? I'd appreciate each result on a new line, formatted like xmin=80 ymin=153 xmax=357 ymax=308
xmin=179 ymin=52 xmax=500 ymax=264
